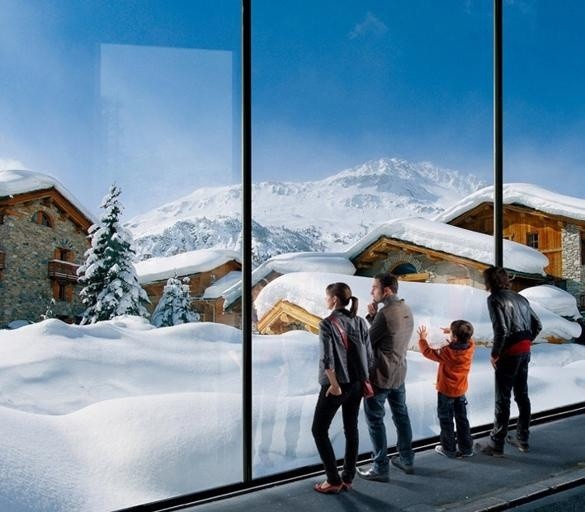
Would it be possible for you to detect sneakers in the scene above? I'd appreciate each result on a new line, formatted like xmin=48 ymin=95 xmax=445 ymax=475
xmin=475 ymin=443 xmax=504 ymax=457
xmin=506 ymin=435 xmax=530 ymax=452
xmin=435 ymin=445 xmax=474 ymax=460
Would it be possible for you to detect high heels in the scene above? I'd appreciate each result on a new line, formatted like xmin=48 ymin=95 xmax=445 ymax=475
xmin=312 ymin=482 xmax=353 ymax=494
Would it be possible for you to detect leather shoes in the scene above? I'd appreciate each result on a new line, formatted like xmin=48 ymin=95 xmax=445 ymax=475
xmin=356 ymin=467 xmax=389 ymax=482
xmin=391 ymin=456 xmax=413 ymax=473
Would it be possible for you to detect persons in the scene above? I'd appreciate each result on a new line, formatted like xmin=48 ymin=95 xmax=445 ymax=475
xmin=355 ymin=272 xmax=414 ymax=482
xmin=312 ymin=283 xmax=375 ymax=494
xmin=417 ymin=320 xmax=475 ymax=459
xmin=476 ymin=266 xmax=543 ymax=458
